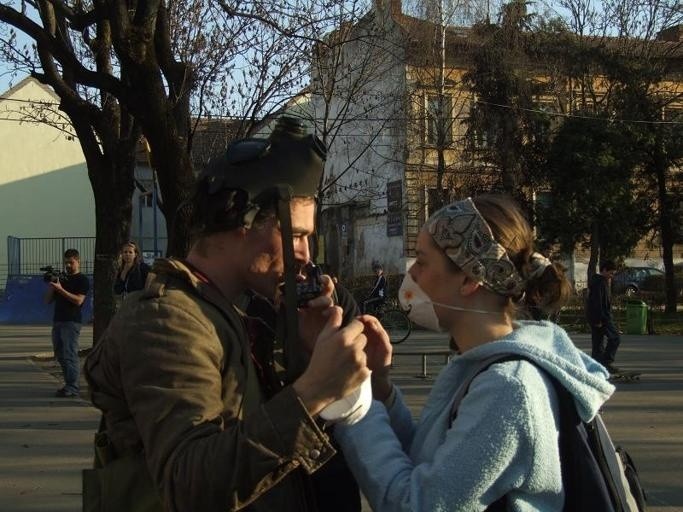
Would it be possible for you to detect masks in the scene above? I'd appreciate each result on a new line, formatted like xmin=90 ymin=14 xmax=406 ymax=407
xmin=397 ymin=272 xmax=469 ymax=334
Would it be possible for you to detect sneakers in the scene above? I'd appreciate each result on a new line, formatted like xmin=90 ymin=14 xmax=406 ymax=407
xmin=55 ymin=384 xmax=80 ymax=397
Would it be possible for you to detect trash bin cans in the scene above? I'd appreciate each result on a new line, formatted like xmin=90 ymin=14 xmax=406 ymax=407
xmin=624 ymin=299 xmax=647 ymax=335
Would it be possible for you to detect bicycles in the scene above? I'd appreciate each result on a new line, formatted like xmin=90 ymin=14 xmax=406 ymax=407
xmin=350 ymin=287 xmax=411 ymax=344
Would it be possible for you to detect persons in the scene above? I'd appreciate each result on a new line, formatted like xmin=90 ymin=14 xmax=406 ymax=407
xmin=43 ymin=248 xmax=91 ymax=399
xmin=109 ymin=240 xmax=152 ymax=314
xmin=586 ymin=260 xmax=621 ymax=378
xmin=293 ymin=190 xmax=648 ymax=512
xmin=75 ymin=115 xmax=394 ymax=511
xmin=359 ymin=263 xmax=387 ymax=317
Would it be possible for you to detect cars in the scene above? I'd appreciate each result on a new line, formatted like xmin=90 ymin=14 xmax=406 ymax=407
xmin=582 ymin=265 xmax=683 ymax=309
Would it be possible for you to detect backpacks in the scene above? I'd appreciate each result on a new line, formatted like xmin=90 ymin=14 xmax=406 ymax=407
xmin=446 ymin=352 xmax=647 ymax=512
